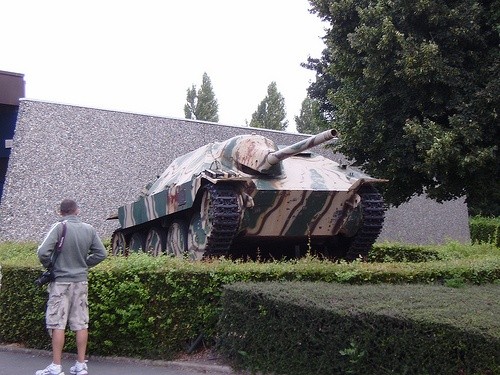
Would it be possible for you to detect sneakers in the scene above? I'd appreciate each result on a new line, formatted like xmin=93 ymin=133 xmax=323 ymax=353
xmin=35 ymin=361 xmax=65 ymax=375
xmin=70 ymin=360 xmax=89 ymax=375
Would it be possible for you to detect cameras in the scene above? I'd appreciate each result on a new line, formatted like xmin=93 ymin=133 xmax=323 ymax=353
xmin=34 ymin=268 xmax=55 ymax=288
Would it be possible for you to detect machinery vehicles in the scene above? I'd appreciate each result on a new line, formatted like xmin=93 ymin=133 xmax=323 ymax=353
xmin=107 ymin=127 xmax=389 ymax=259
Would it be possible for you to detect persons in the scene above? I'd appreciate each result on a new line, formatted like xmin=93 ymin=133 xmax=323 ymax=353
xmin=35 ymin=199 xmax=107 ymax=375
